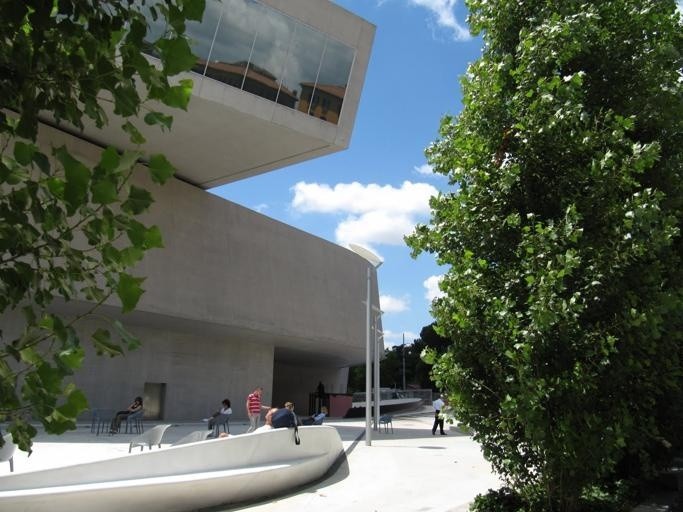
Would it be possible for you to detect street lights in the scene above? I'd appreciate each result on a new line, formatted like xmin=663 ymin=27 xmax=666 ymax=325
xmin=349 ymin=243 xmax=385 ymax=446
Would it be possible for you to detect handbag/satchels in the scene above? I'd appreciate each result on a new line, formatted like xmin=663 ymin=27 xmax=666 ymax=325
xmin=272 ymin=409 xmax=298 ymax=429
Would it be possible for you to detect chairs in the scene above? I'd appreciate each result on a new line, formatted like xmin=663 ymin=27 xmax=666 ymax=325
xmin=129 ymin=424 xmax=172 ymax=453
xmin=209 ymin=415 xmax=230 ymax=436
xmin=379 ymin=415 xmax=394 ymax=434
xmin=0 ymin=432 xmax=16 ymax=472
xmin=91 ymin=406 xmax=145 ymax=436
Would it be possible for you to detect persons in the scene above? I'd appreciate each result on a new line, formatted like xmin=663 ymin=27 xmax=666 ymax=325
xmin=284 ymin=401 xmax=298 ymax=426
xmin=311 ymin=406 xmax=328 ymax=425
xmin=207 ymin=397 xmax=232 ymax=436
xmin=314 ymin=379 xmax=324 ymax=397
xmin=244 ymin=387 xmax=271 ymax=434
xmin=218 ymin=406 xmax=279 ymax=438
xmin=108 ymin=396 xmax=142 ymax=433
xmin=430 ymin=393 xmax=448 ymax=435
